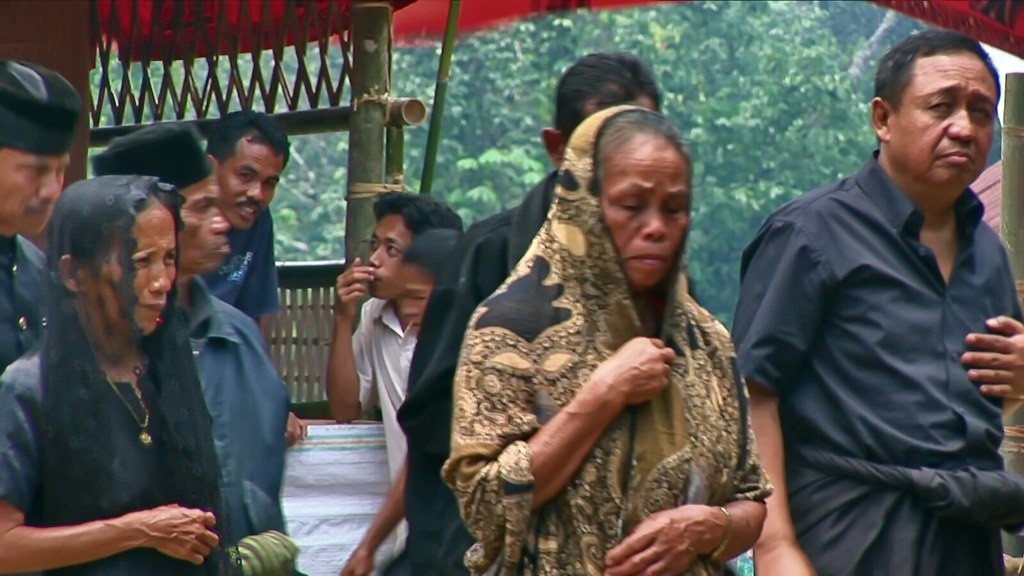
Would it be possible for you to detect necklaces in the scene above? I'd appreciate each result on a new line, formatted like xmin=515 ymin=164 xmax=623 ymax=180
xmin=96 ymin=362 xmax=152 ymax=446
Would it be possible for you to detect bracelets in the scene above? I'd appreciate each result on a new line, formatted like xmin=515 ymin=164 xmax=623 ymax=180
xmin=708 ymin=505 xmax=732 ymax=561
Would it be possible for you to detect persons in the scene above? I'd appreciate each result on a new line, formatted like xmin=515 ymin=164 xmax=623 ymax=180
xmin=0 ymin=58 xmax=308 ymax=576
xmin=326 ymin=33 xmax=1024 ymax=576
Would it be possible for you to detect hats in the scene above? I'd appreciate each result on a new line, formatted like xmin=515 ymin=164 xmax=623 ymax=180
xmin=91 ymin=123 xmax=212 ymax=192
xmin=0 ymin=58 xmax=82 ymax=153
